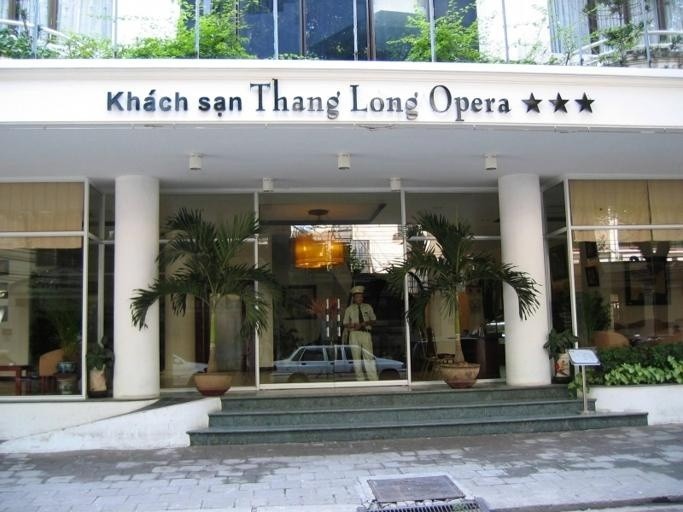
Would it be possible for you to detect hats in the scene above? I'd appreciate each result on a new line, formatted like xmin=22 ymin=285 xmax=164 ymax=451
xmin=160 ymin=350 xmax=208 ymax=388
xmin=270 ymin=344 xmax=407 ymax=384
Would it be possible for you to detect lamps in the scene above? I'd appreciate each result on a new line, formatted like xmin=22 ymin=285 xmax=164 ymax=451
xmin=589 ymin=331 xmax=631 ymax=347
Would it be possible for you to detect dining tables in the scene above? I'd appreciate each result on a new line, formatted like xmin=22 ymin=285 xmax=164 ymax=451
xmin=29 ymin=264 xmax=82 ymax=394
xmin=86 ymin=337 xmax=115 ymax=392
xmin=378 ymin=207 xmax=579 ymax=389
xmin=130 ymin=205 xmax=284 ymax=395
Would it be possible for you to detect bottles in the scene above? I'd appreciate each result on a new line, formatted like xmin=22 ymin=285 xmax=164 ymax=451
xmin=352 ymin=285 xmax=366 ymax=295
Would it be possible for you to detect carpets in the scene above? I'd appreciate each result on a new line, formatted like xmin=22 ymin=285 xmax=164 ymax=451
xmin=357 ymin=304 xmax=365 ymax=332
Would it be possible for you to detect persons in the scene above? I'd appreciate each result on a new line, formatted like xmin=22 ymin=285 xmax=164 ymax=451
xmin=342 ymin=285 xmax=379 ymax=381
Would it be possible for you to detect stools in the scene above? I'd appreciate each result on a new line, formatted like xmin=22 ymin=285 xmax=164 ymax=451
xmin=358 ymin=328 xmax=371 ymax=332
